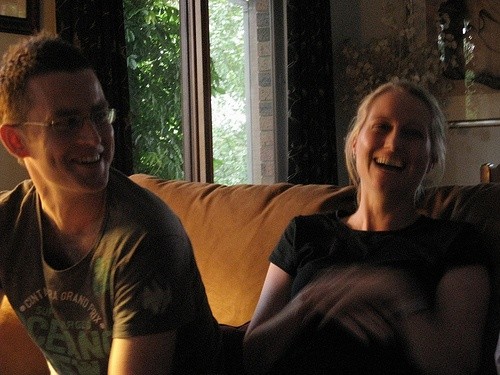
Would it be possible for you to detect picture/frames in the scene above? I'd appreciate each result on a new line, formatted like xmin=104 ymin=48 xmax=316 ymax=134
xmin=0 ymin=0 xmax=42 ymax=36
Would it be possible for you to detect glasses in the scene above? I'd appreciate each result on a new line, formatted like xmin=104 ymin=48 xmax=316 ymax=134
xmin=5 ymin=108 xmax=118 ymax=133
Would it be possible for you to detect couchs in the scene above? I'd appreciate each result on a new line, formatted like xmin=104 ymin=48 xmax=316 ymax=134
xmin=0 ymin=174 xmax=500 ymax=375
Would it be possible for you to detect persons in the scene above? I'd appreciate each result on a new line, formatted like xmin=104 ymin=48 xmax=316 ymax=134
xmin=1 ymin=30 xmax=217 ymax=375
xmin=243 ymin=78 xmax=500 ymax=375
